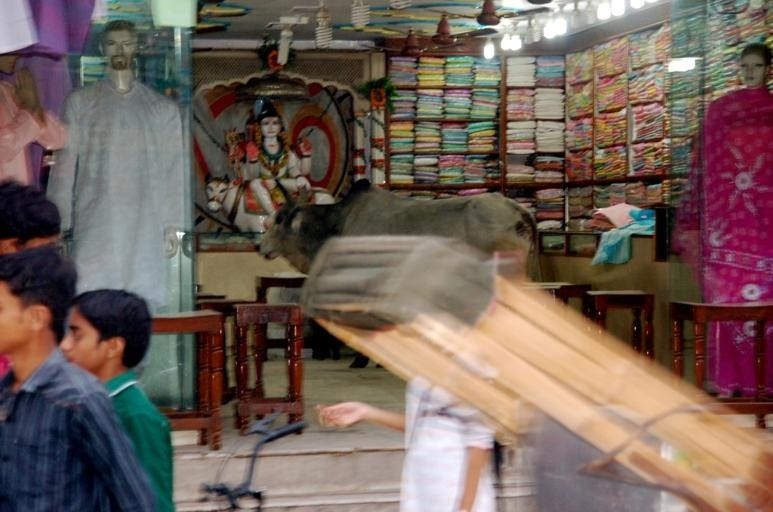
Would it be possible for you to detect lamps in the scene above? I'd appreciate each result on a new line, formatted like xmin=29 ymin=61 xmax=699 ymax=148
xmin=262 ymin=2 xmax=460 ymax=65
xmin=475 ymin=0 xmax=687 ymax=60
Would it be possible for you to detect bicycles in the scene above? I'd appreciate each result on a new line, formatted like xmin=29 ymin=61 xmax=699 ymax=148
xmin=201 ymin=407 xmax=313 ymax=512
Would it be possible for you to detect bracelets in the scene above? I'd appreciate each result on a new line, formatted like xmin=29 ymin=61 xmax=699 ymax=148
xmin=300 ymin=154 xmax=311 ymax=158
xmin=247 ymin=160 xmax=258 ymax=163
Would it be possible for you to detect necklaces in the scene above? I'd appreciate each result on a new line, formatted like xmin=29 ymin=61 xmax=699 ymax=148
xmin=263 ymin=141 xmax=278 ymax=147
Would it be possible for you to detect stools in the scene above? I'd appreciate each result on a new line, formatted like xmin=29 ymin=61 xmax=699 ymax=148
xmin=253 ymin=272 xmax=326 ymax=365
xmin=537 ymin=266 xmax=587 ymax=337
xmin=671 ymin=297 xmax=771 ymax=433
xmin=206 ymin=288 xmax=307 ymax=433
xmin=141 ymin=305 xmax=224 ymax=455
xmin=584 ymin=283 xmax=657 ymax=361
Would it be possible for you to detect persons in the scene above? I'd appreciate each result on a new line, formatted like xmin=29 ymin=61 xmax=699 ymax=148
xmin=0 ymin=245 xmax=161 ymax=512
xmin=311 ymin=379 xmax=499 ymax=512
xmin=0 ymin=178 xmax=64 ymax=377
xmin=225 ymin=95 xmax=314 ymax=231
xmin=44 ymin=15 xmax=188 ymax=407
xmin=59 ymin=285 xmax=180 ymax=512
xmin=0 ymin=53 xmax=69 ymax=195
xmin=666 ymin=43 xmax=772 ymax=400
